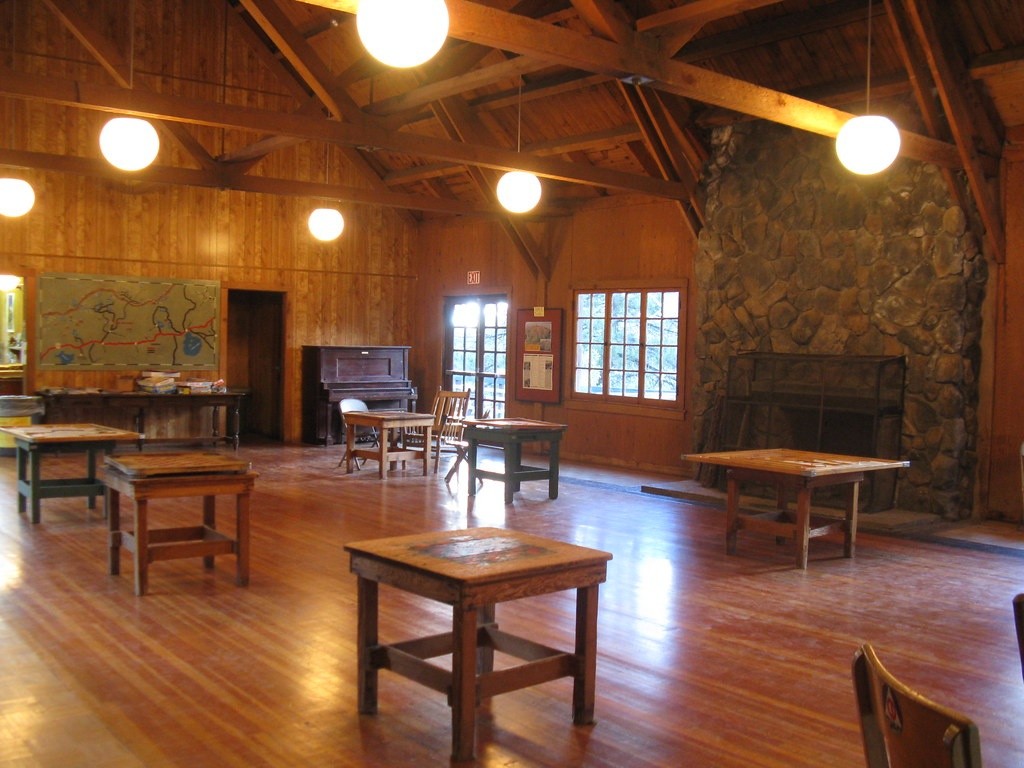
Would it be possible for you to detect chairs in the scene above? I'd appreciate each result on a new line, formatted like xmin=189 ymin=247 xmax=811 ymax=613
xmin=401 ymin=386 xmax=471 ymax=473
xmin=338 ymin=398 xmax=380 ymax=471
xmin=850 ymin=641 xmax=983 ymax=768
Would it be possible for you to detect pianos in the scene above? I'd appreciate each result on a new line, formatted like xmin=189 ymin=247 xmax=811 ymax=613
xmin=302 ymin=345 xmax=419 ymax=447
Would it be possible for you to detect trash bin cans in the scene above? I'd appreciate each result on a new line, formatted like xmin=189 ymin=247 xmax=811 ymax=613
xmin=1 ymin=395 xmax=46 ymax=457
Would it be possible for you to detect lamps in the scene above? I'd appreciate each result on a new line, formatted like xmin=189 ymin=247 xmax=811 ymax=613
xmin=464 ymin=426 xmax=568 ymax=503
xmin=0 ymin=0 xmax=902 ymax=243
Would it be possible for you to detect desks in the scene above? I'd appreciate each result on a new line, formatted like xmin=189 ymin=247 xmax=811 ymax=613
xmin=36 ymin=390 xmax=245 ymax=454
xmin=12 ymin=437 xmax=117 ymax=524
xmin=680 ymin=447 xmax=909 ymax=570
xmin=342 ymin=526 xmax=612 ymax=760
xmin=343 ymin=408 xmax=436 ymax=480
xmin=97 ymin=464 xmax=261 ymax=595
